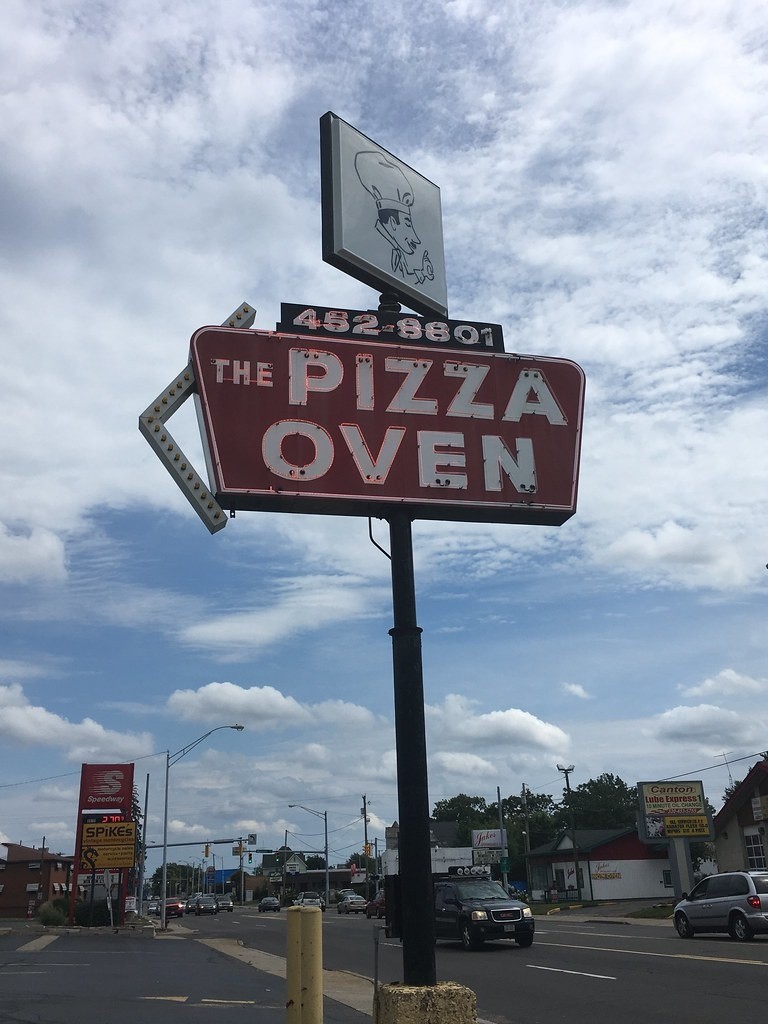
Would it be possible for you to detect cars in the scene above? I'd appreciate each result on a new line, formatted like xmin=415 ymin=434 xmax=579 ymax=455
xmin=366 ymin=890 xmax=385 ymax=918
xmin=319 ymin=896 xmax=326 ymax=911
xmin=337 ymin=889 xmax=357 ymax=903
xmin=337 ymin=895 xmax=367 ymax=914
xmin=258 ymin=896 xmax=280 ymax=913
xmin=147 ymin=895 xmax=234 ymax=919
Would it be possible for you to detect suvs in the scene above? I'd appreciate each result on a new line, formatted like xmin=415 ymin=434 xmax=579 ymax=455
xmin=291 ymin=891 xmax=322 ymax=909
xmin=386 ymin=865 xmax=536 ymax=951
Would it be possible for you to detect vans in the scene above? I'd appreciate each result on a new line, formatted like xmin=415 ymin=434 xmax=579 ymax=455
xmin=673 ymin=870 xmax=768 ymax=942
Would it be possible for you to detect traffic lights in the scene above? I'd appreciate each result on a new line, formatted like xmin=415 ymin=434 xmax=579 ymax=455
xmin=248 ymin=853 xmax=253 ymax=864
xmin=275 ymin=852 xmax=280 ymax=862
xmin=362 ymin=844 xmax=374 ymax=856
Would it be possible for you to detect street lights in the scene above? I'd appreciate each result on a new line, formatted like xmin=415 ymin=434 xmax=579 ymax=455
xmin=556 ymin=763 xmax=582 ymax=904
xmin=161 ymin=724 xmax=245 ymax=929
xmin=288 ymin=804 xmax=329 ymax=905
xmin=134 ymin=840 xmax=155 ymax=898
xmin=179 ymin=850 xmax=224 ymax=894
